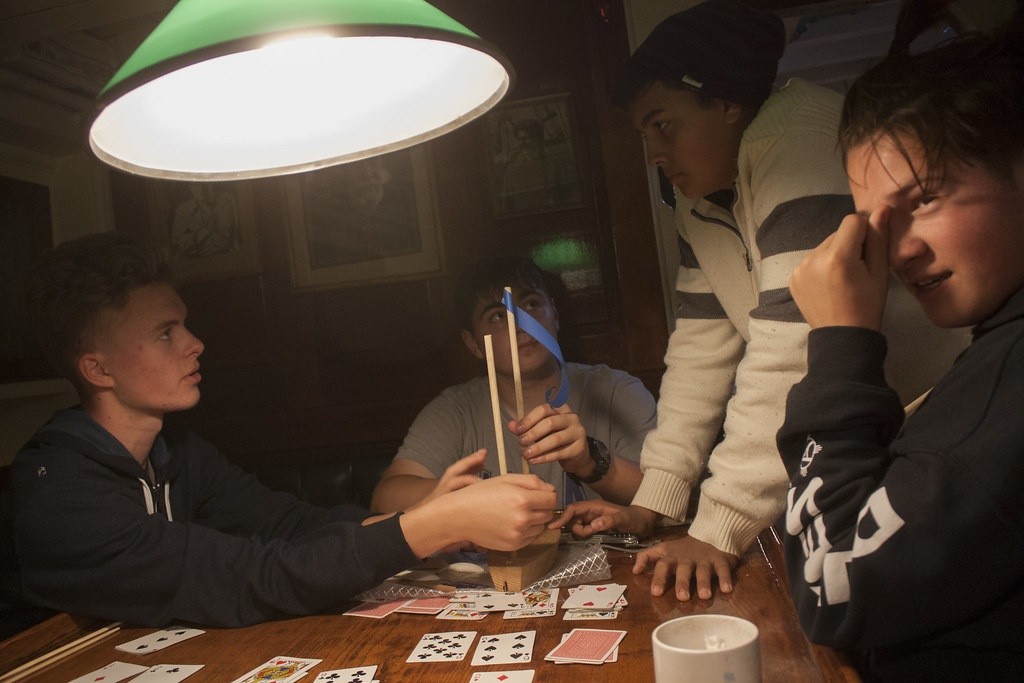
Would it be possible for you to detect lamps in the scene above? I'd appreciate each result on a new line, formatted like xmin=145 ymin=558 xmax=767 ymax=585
xmin=87 ymin=0 xmax=519 ymax=182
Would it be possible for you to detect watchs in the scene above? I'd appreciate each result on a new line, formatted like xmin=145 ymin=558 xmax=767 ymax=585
xmin=561 ymin=435 xmax=611 ymax=486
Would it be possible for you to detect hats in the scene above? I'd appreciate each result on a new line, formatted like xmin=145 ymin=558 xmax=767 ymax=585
xmin=614 ymin=0 xmax=785 ymax=104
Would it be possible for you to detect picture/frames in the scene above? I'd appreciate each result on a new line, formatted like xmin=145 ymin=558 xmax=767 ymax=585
xmin=137 ymin=175 xmax=263 ymax=289
xmin=279 ymin=141 xmax=455 ymax=295
xmin=526 ymin=229 xmax=614 ymax=340
xmin=480 ymin=90 xmax=590 ymax=224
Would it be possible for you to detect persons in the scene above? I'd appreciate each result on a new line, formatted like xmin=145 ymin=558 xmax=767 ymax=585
xmin=371 ymin=250 xmax=661 ymax=570
xmin=773 ymin=34 xmax=1024 ymax=682
xmin=553 ymin=0 xmax=978 ymax=604
xmin=1 ymin=232 xmax=562 ymax=642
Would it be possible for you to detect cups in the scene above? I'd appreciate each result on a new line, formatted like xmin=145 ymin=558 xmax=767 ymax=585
xmin=648 ymin=612 xmax=761 ymax=682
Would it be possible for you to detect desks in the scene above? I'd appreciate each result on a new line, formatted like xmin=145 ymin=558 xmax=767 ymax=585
xmin=0 ymin=515 xmax=866 ymax=683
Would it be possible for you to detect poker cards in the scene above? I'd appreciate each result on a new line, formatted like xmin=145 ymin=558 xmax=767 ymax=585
xmin=68 ymin=579 xmax=628 ymax=682
